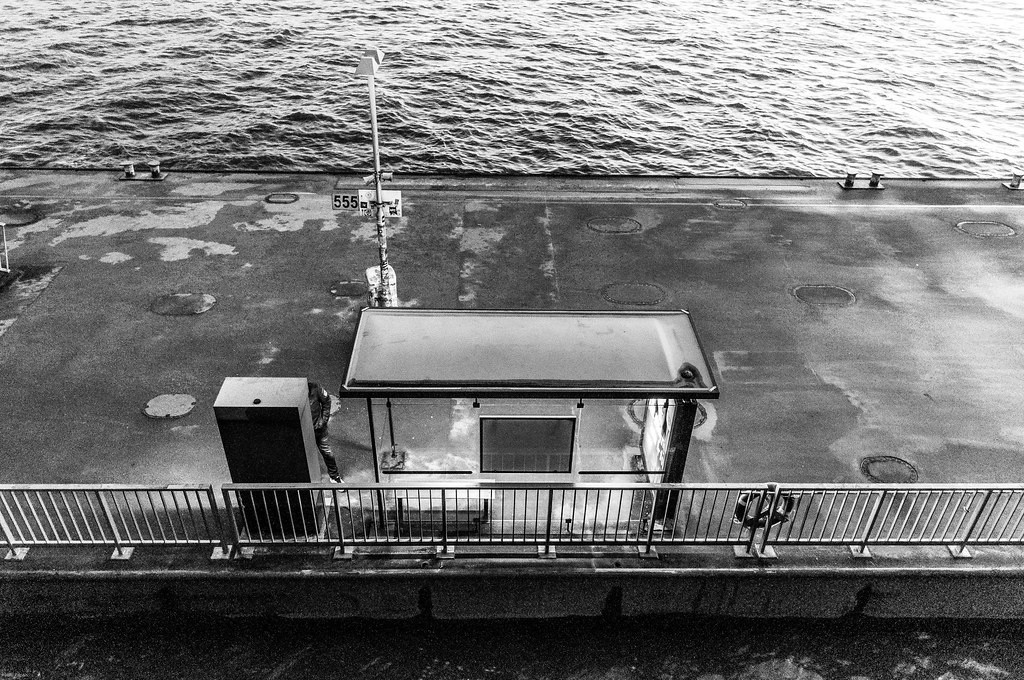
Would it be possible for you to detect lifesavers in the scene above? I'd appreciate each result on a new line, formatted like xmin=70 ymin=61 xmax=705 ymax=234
xmin=736 ymin=490 xmax=795 ymax=529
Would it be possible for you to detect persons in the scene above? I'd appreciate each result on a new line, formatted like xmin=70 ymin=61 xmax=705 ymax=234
xmin=308 ymin=382 xmax=347 ymax=493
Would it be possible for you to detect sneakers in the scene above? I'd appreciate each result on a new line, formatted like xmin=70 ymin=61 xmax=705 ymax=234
xmin=330 ymin=475 xmax=349 ymax=492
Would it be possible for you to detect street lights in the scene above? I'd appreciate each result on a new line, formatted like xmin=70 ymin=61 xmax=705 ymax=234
xmin=355 ymin=45 xmax=391 ymax=308
